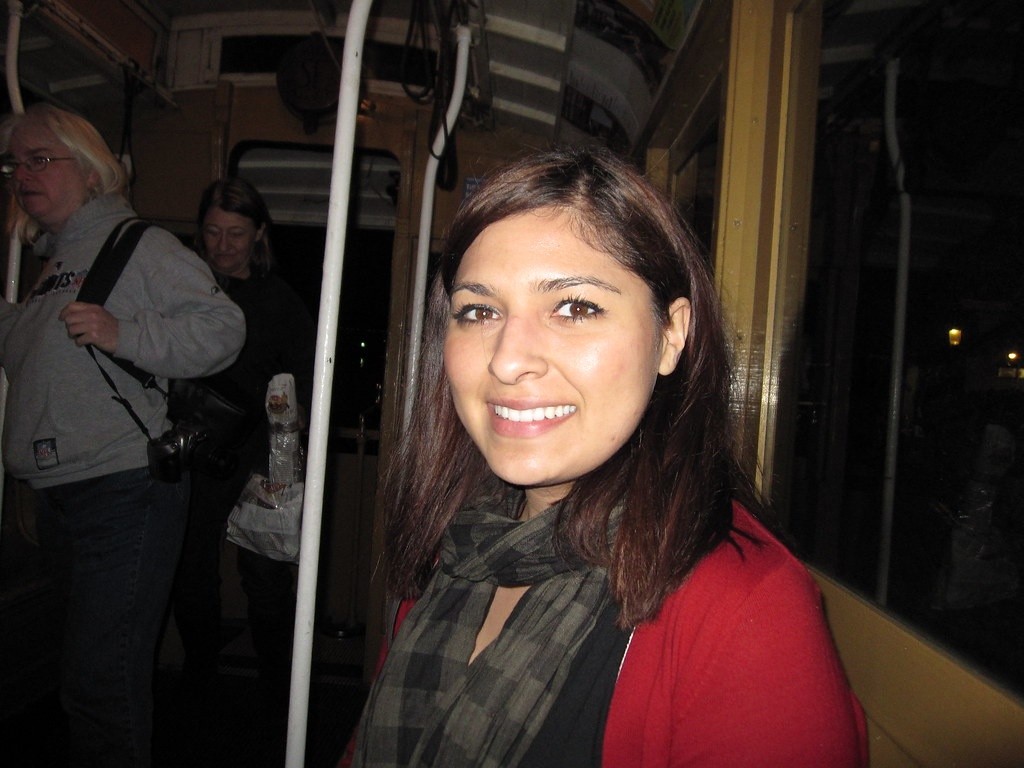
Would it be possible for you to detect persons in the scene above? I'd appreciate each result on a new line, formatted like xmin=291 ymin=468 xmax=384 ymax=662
xmin=338 ymin=149 xmax=869 ymax=768
xmin=0 ymin=104 xmax=244 ymax=768
xmin=176 ymin=178 xmax=310 ymax=706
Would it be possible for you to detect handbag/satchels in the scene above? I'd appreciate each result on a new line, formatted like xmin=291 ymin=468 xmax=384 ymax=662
xmin=167 ymin=385 xmax=248 ymax=465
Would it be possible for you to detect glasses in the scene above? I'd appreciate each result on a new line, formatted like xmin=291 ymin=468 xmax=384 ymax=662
xmin=0 ymin=155 xmax=74 ymax=179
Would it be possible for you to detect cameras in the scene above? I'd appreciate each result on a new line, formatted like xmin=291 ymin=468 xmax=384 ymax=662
xmin=146 ymin=415 xmax=234 ymax=483
